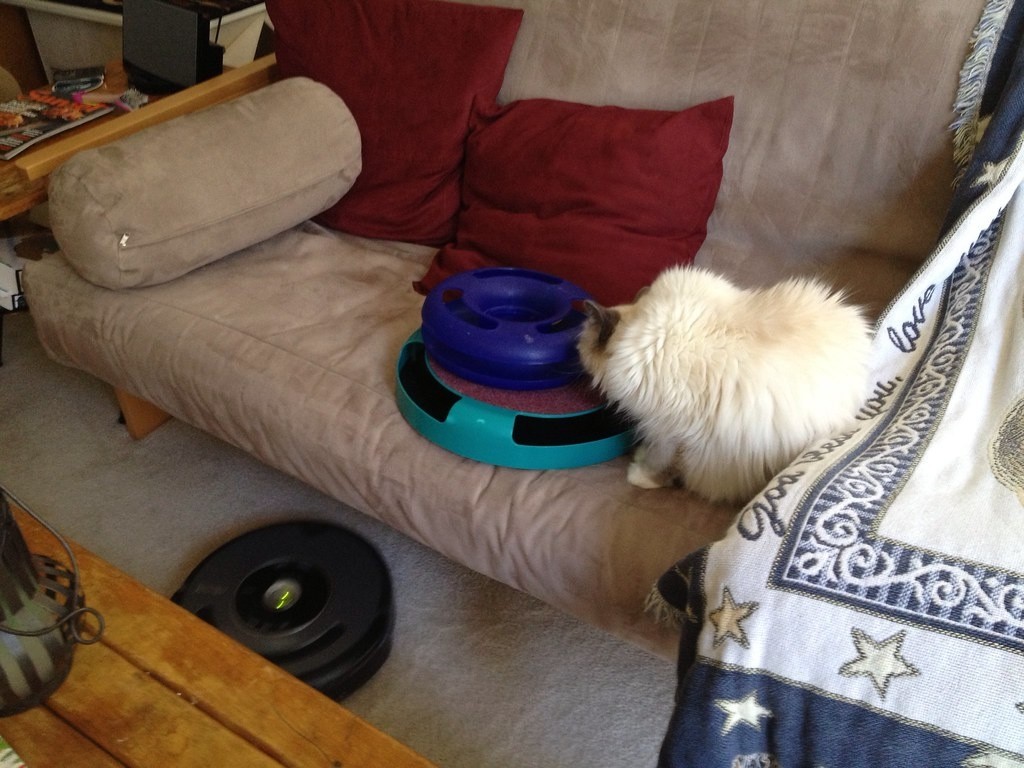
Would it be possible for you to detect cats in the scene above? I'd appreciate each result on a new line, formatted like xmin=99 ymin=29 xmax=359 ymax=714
xmin=574 ymin=261 xmax=875 ymax=507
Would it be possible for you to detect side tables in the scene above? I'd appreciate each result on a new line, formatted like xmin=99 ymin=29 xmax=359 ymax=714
xmin=0 ymin=57 xmax=239 ymax=221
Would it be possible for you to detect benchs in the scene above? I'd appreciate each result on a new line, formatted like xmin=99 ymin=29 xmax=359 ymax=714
xmin=14 ymin=0 xmax=1024 ymax=662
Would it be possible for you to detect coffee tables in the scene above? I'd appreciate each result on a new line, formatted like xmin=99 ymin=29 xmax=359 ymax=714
xmin=0 ymin=502 xmax=442 ymax=768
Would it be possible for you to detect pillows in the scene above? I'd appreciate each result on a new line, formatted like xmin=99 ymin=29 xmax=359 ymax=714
xmin=265 ymin=0 xmax=523 ymax=249
xmin=50 ymin=77 xmax=364 ymax=290
xmin=413 ymin=94 xmax=735 ymax=300
